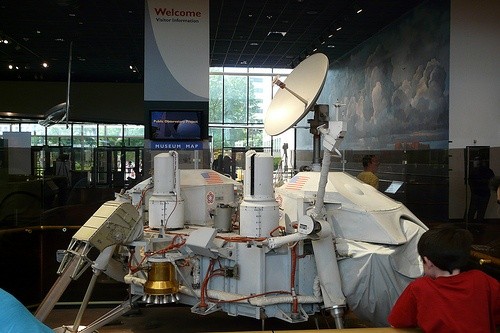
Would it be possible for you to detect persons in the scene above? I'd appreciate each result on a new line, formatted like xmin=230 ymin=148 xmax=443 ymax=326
xmin=466 ymin=153 xmax=497 ymax=221
xmin=212 ymin=155 xmax=231 ymax=175
xmin=386 ymin=226 xmax=500 ymax=333
xmin=489 ymin=176 xmax=500 ymax=205
xmin=355 ymin=154 xmax=379 ymax=191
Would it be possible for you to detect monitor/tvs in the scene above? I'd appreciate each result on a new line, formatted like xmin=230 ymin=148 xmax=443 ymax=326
xmin=149 ymin=109 xmax=205 ymax=140
